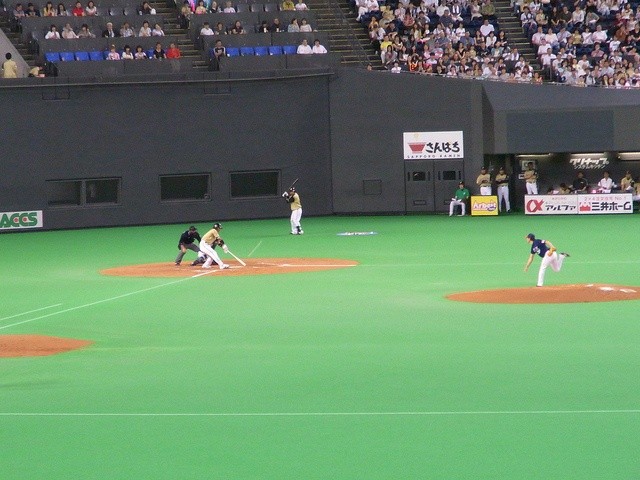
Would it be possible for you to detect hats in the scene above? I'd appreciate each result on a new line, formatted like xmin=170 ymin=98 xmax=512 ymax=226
xmin=499 ymin=166 xmax=504 ymax=171
xmin=459 ymin=181 xmax=463 ymax=185
xmin=189 ymin=225 xmax=197 ymax=231
xmin=626 ymin=171 xmax=630 ymax=174
xmin=481 ymin=166 xmax=486 ymax=170
xmin=524 ymin=233 xmax=535 ymax=238
xmin=528 ymin=162 xmax=533 ymax=164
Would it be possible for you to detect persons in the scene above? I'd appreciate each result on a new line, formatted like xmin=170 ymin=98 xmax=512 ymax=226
xmin=2 ymin=52 xmax=17 ymax=78
xmin=259 ymin=20 xmax=271 ymax=32
xmin=573 ymin=172 xmax=588 ymax=193
xmin=287 ymin=18 xmax=300 ymax=32
xmin=546 ymin=188 xmax=559 ymax=195
xmin=139 ymin=1 xmax=152 ymax=15
xmin=475 ymin=166 xmax=493 ymax=196
xmin=56 ymin=3 xmax=70 ymax=16
xmin=173 ymin=225 xmax=201 ymax=266
xmin=43 ymin=1 xmax=55 ymax=17
xmin=209 ymin=0 xmax=222 ymax=13
xmin=85 ymin=1 xmax=98 ymax=17
xmin=44 ymin=24 xmax=61 ymax=39
xmin=107 ymin=47 xmax=120 ymax=60
xmin=280 ymin=0 xmax=295 ymax=11
xmin=295 ymin=0 xmax=310 ymax=11
xmin=151 ymin=23 xmax=165 ymax=36
xmin=223 ymin=1 xmax=236 ymax=13
xmin=195 ymin=0 xmax=207 ymax=14
xmin=448 ymin=181 xmax=469 ymax=217
xmin=166 ymin=42 xmax=180 ymax=59
xmin=72 ymin=1 xmax=86 ymax=17
xmin=212 ymin=39 xmax=226 ymax=58
xmin=353 ymin=0 xmax=543 ymax=85
xmin=11 ymin=3 xmax=25 ymax=20
xmin=300 ymin=18 xmax=312 ymax=32
xmin=282 ymin=187 xmax=304 ymax=235
xmin=523 ymin=233 xmax=570 ymax=287
xmin=28 ymin=64 xmax=43 ymax=78
xmin=138 ymin=21 xmax=151 ymax=37
xmin=134 ymin=45 xmax=148 ymax=60
xmin=559 ymin=183 xmax=573 ymax=194
xmin=271 ymin=18 xmax=286 ymax=32
xmin=199 ymin=222 xmax=230 ymax=270
xmin=119 ymin=21 xmax=133 ymax=37
xmin=312 ymin=39 xmax=328 ymax=54
xmin=621 ymin=170 xmax=635 ymax=193
xmin=509 ymin=0 xmax=640 ymax=90
xmin=495 ymin=166 xmax=512 ymax=213
xmin=120 ymin=44 xmax=133 ymax=60
xmin=597 ymin=171 xmax=617 ymax=194
xmin=199 ymin=22 xmax=214 ymax=35
xmin=76 ymin=23 xmax=96 ymax=39
xmin=214 ymin=22 xmax=227 ymax=35
xmin=296 ymin=39 xmax=312 ymax=55
xmin=524 ymin=161 xmax=539 ymax=194
xmin=61 ymin=23 xmax=77 ymax=39
xmin=181 ymin=1 xmax=191 ymax=18
xmin=232 ymin=20 xmax=244 ymax=35
xmin=191 ymin=242 xmax=218 ymax=266
xmin=153 ymin=41 xmax=167 ymax=59
xmin=632 ymin=175 xmax=640 ymax=201
xmin=102 ymin=22 xmax=121 ymax=38
xmin=25 ymin=3 xmax=41 ymax=18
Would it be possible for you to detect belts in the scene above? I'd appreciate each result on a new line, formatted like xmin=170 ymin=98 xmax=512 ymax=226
xmin=526 ymin=181 xmax=536 ymax=183
xmin=482 ymin=186 xmax=491 ymax=187
xmin=498 ymin=185 xmax=507 ymax=187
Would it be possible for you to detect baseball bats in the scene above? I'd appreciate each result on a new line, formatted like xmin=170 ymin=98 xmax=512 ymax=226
xmin=286 ymin=179 xmax=298 ymax=192
xmin=227 ymin=250 xmax=246 ymax=267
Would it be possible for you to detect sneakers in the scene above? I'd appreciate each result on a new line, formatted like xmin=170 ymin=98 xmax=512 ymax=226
xmin=449 ymin=213 xmax=453 ymax=216
xmin=290 ymin=232 xmax=297 ymax=234
xmin=298 ymin=231 xmax=304 ymax=234
xmin=560 ymin=253 xmax=571 ymax=257
xmin=220 ymin=264 xmax=230 ymax=270
xmin=507 ymin=209 xmax=512 ymax=215
xmin=175 ymin=262 xmax=180 ymax=266
xmin=202 ymin=264 xmax=212 ymax=268
xmin=498 ymin=211 xmax=502 ymax=214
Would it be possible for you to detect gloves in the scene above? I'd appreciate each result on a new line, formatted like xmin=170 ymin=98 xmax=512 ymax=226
xmin=285 ymin=192 xmax=289 ymax=197
xmin=222 ymin=244 xmax=228 ymax=251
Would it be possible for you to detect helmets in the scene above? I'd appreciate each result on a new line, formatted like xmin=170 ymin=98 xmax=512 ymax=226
xmin=213 ymin=223 xmax=223 ymax=230
xmin=289 ymin=187 xmax=295 ymax=192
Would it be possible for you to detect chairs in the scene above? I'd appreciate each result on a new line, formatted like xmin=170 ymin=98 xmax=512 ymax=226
xmin=122 ymin=7 xmax=137 ymax=16
xmin=119 ymin=49 xmax=134 ymax=59
xmin=75 ymin=51 xmax=90 ymax=61
xmin=284 ymin=45 xmax=298 ymax=55
xmin=31 ymin=30 xmax=43 ymax=42
xmin=463 ymin=15 xmax=498 ymax=25
xmin=255 ymin=25 xmax=261 ymax=32
xmin=235 ymin=3 xmax=250 ymax=13
xmin=242 ymin=24 xmax=255 ymax=32
xmin=574 ymin=178 xmax=588 ymax=194
xmin=59 ymin=51 xmax=76 ymax=62
xmin=109 ymin=7 xmax=124 ymax=16
xmin=44 ymin=51 xmax=62 ymax=62
xmin=454 ymin=192 xmax=471 ymax=216
xmin=264 ymin=3 xmax=278 ymax=13
xmin=134 ymin=49 xmax=148 ymax=59
xmin=209 ymin=48 xmax=227 ymax=63
xmin=251 ymin=3 xmax=264 ymax=13
xmin=89 ymin=51 xmax=104 ymax=60
xmin=466 ymin=27 xmax=499 ymax=36
xmin=226 ymin=48 xmax=240 ymax=58
xmin=148 ymin=49 xmax=155 ymax=59
xmin=104 ymin=50 xmax=122 ymax=59
xmin=240 ymin=46 xmax=255 ymax=57
xmin=268 ymin=46 xmax=283 ymax=56
xmin=575 ymin=45 xmax=591 ymax=55
xmin=254 ymin=46 xmax=269 ymax=56
